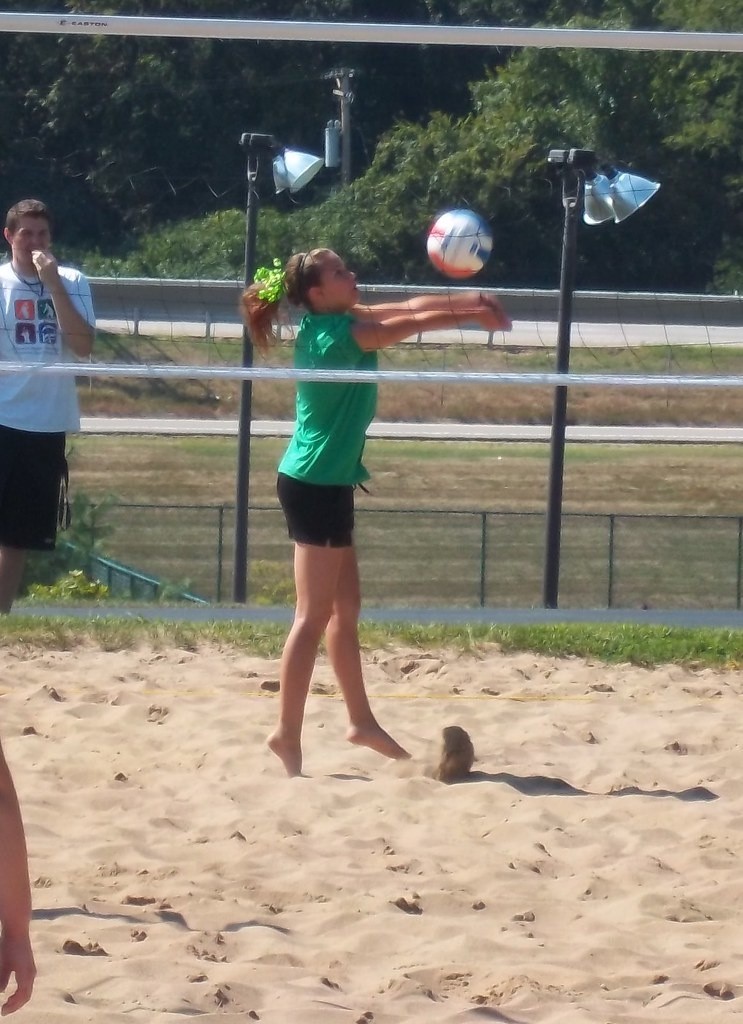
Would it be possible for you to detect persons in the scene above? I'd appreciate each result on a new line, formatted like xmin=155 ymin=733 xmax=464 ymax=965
xmin=0 ymin=739 xmax=36 ymax=1016
xmin=240 ymin=249 xmax=512 ymax=779
xmin=0 ymin=198 xmax=97 ymax=620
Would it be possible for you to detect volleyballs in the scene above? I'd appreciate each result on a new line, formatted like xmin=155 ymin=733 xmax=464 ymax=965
xmin=426 ymin=209 xmax=494 ymax=280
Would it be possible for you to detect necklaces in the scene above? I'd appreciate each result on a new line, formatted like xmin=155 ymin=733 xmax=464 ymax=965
xmin=10 ymin=261 xmax=45 ymax=296
